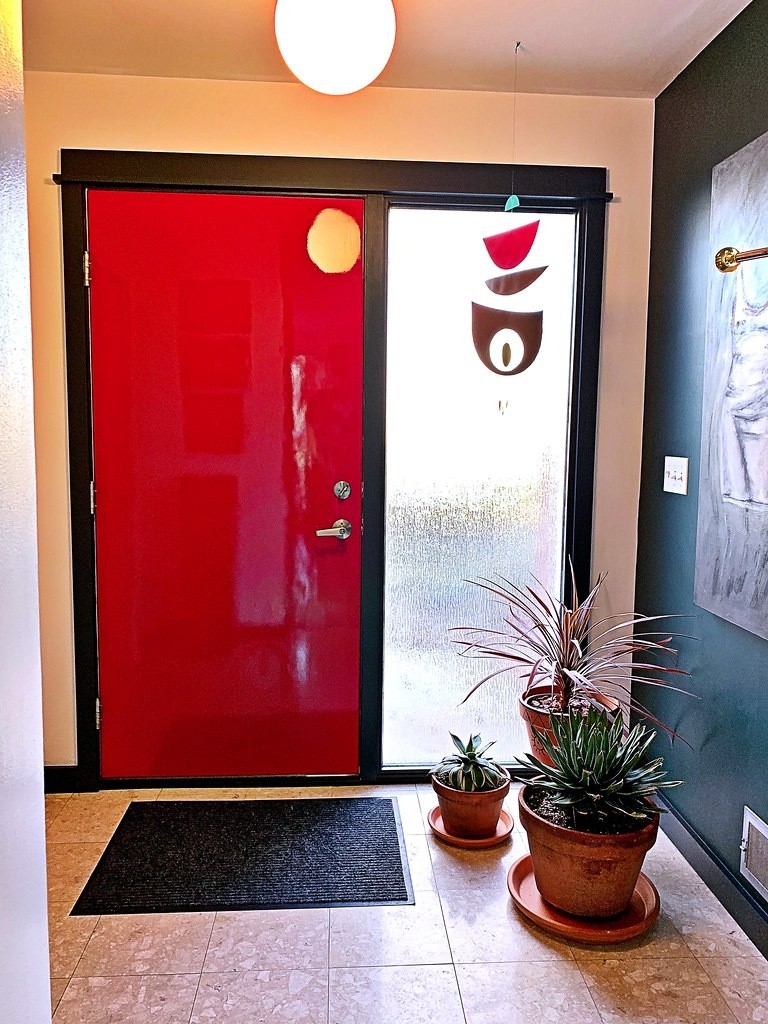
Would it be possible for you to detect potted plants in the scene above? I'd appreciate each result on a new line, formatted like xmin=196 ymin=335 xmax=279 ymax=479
xmin=429 ymin=729 xmax=515 ymax=838
xmin=509 ymin=700 xmax=687 ymax=920
xmin=446 ymin=554 xmax=703 ymax=769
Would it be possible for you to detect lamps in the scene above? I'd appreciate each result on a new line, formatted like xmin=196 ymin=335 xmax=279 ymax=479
xmin=274 ymin=0 xmax=395 ymax=97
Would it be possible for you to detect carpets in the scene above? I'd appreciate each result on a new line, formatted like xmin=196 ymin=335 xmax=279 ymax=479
xmin=70 ymin=797 xmax=416 ymax=916
